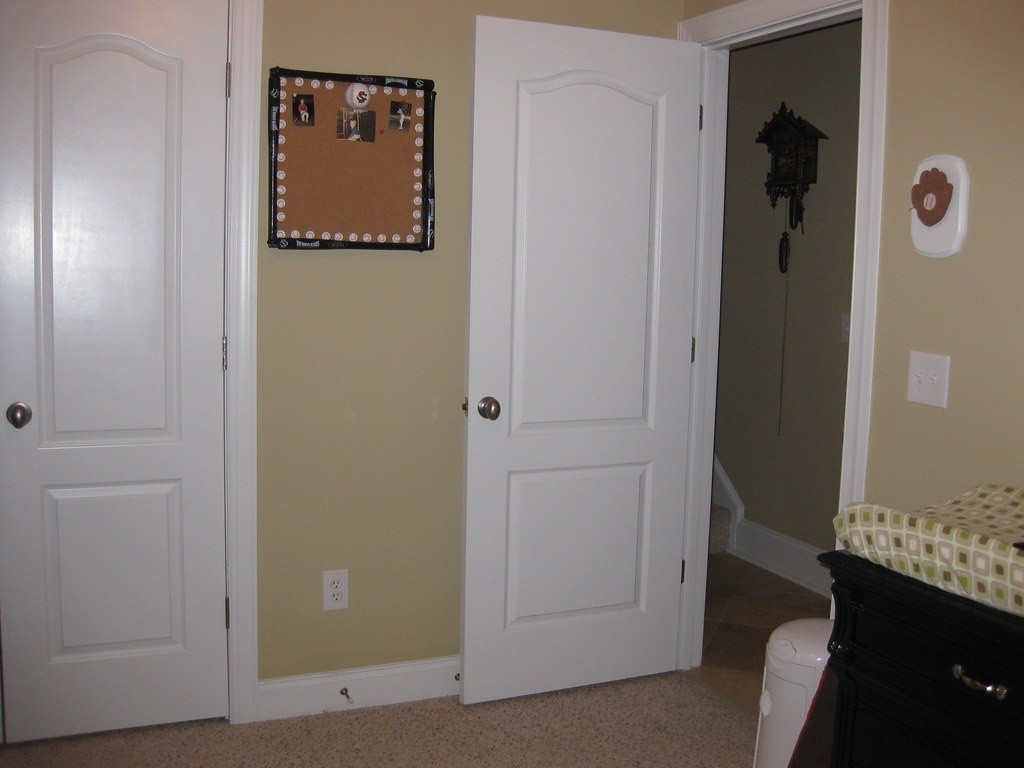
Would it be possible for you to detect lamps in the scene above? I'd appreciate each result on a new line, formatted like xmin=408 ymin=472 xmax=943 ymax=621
xmin=755 ymin=101 xmax=831 ymax=274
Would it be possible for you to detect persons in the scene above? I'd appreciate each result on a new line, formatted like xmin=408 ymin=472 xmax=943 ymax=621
xmin=397 ymin=109 xmax=405 ymax=129
xmin=299 ymin=100 xmax=309 ymax=124
xmin=347 ymin=118 xmax=360 ymax=141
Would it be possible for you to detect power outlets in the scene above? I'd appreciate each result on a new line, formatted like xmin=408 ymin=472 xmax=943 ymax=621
xmin=323 ymin=567 xmax=350 ymax=611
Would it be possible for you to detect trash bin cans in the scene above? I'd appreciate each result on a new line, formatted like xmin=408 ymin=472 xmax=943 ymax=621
xmin=752 ymin=618 xmax=835 ymax=768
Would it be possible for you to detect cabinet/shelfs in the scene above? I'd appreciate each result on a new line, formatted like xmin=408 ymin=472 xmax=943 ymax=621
xmin=816 ymin=549 xmax=1024 ymax=768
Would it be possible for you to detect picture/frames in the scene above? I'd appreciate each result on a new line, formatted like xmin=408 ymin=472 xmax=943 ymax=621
xmin=266 ymin=65 xmax=436 ymax=254
